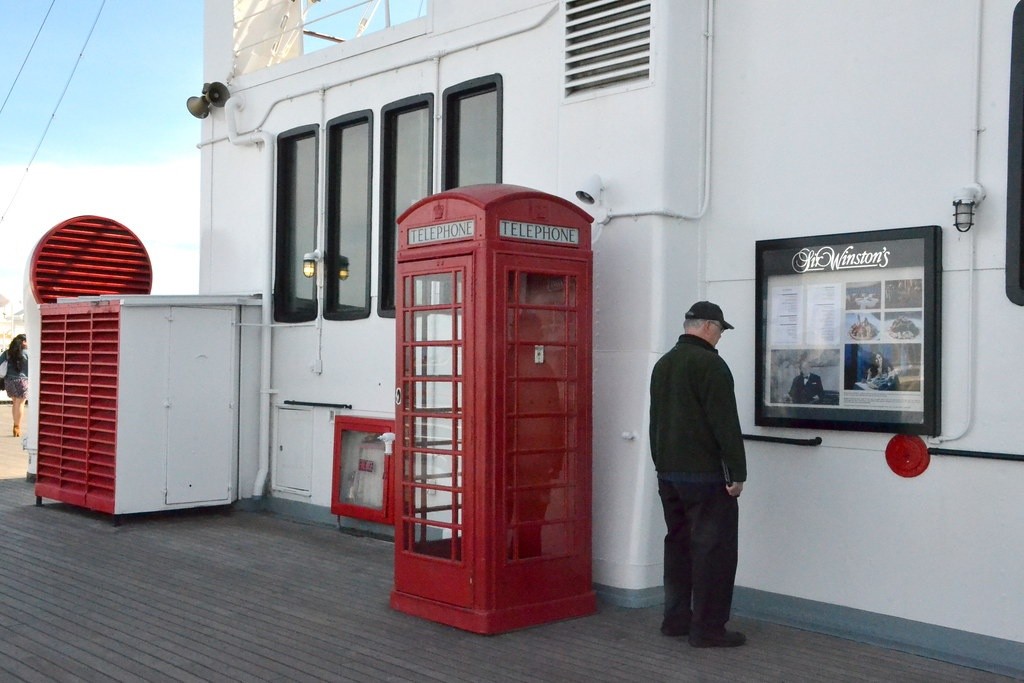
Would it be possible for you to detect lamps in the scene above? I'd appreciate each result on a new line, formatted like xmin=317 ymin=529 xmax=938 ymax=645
xmin=951 ymin=184 xmax=987 ymax=232
xmin=304 ymin=250 xmax=321 ymax=278
xmin=338 ymin=255 xmax=350 ymax=281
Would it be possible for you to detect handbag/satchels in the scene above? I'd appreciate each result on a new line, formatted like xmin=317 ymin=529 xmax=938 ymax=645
xmin=0 ymin=378 xmax=5 ymax=391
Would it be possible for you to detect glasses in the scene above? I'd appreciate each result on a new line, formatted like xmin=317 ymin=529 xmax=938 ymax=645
xmin=709 ymin=320 xmax=725 ymax=333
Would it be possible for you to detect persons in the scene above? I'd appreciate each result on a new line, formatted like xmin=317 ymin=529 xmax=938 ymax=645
xmin=0 ymin=334 xmax=28 ymax=437
xmin=784 ymin=361 xmax=825 ymax=404
xmin=865 ymin=351 xmax=900 ymax=391
xmin=649 ymin=300 xmax=748 ymax=648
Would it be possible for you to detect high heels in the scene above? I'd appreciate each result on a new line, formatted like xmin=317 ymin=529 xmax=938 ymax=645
xmin=13 ymin=426 xmax=21 ymax=437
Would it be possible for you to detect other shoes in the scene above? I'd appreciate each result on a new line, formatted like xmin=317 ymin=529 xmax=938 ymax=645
xmin=661 ymin=617 xmax=746 ymax=648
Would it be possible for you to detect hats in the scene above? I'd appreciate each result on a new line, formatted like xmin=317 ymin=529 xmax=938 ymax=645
xmin=685 ymin=301 xmax=734 ymax=330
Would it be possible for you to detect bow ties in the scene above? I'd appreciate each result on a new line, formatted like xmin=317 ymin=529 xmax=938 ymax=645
xmin=803 ymin=376 xmax=808 ymax=379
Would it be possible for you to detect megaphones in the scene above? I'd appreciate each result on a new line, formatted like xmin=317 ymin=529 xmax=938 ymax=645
xmin=205 ymin=82 xmax=230 ymax=108
xmin=575 ymin=174 xmax=602 ymax=205
xmin=187 ymin=96 xmax=209 ymax=119
